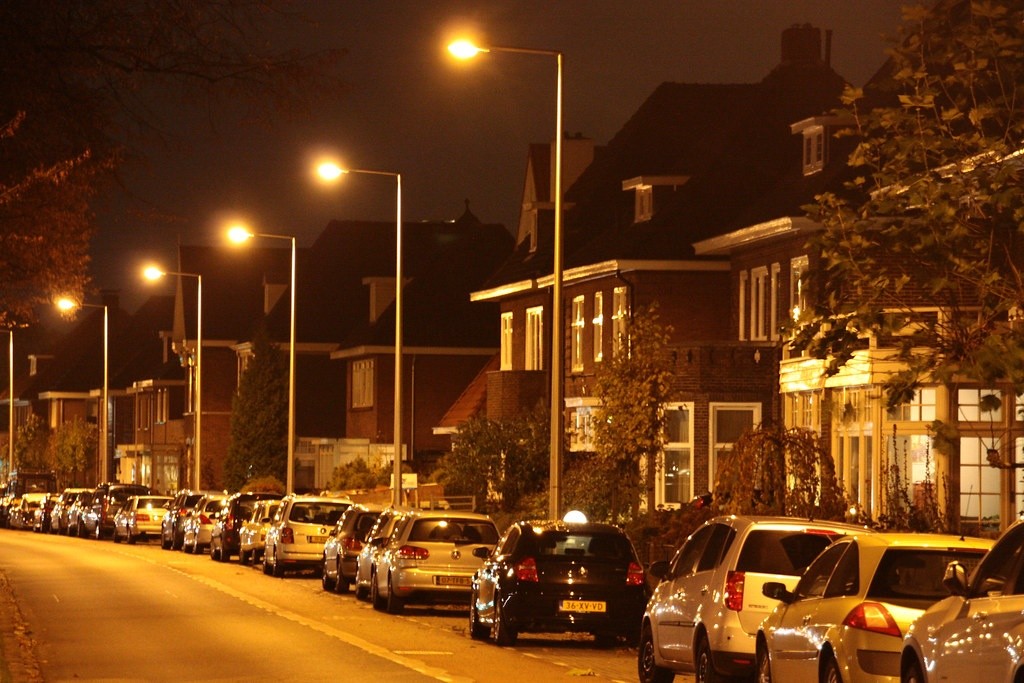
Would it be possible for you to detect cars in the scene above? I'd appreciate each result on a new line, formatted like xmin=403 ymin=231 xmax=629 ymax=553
xmin=898 ymin=515 xmax=1024 ymax=683
xmin=754 ymin=531 xmax=999 ymax=683
xmin=319 ymin=503 xmax=502 ymax=615
xmin=0 ymin=480 xmax=315 ymax=567
xmin=468 ymin=520 xmax=653 ymax=648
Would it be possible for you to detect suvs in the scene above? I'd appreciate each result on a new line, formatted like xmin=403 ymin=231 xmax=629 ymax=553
xmin=260 ymin=492 xmax=356 ymax=578
xmin=637 ymin=514 xmax=883 ymax=683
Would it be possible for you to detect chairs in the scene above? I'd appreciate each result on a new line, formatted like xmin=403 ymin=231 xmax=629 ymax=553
xmin=565 ymin=548 xmax=584 ymax=556
xmin=587 ymin=536 xmax=614 ymax=557
xmin=536 ymin=539 xmax=557 ymax=557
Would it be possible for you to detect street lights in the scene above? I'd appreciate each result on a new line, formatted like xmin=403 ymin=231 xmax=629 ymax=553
xmin=449 ymin=38 xmax=563 ymax=523
xmin=227 ymin=224 xmax=297 ymax=498
xmin=315 ymin=159 xmax=403 ymax=511
xmin=58 ymin=297 xmax=108 ymax=485
xmin=145 ymin=266 xmax=203 ymax=490
